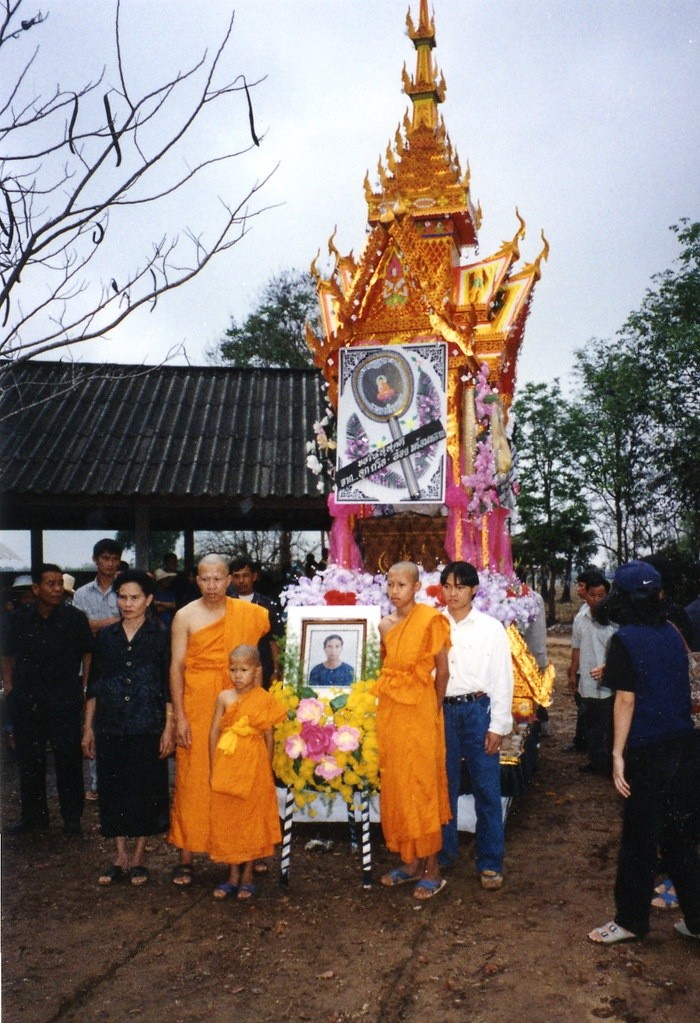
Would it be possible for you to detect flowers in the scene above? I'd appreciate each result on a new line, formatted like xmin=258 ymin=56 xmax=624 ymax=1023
xmin=461 ymin=361 xmax=500 ymax=518
xmin=304 ymin=381 xmax=336 ymax=497
xmin=268 ymin=556 xmax=542 ymax=633
xmin=267 ymin=678 xmax=384 ymax=821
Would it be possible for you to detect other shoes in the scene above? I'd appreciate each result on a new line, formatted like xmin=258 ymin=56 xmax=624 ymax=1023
xmin=7 ymin=732 xmax=16 ymax=750
xmin=480 ymin=870 xmax=503 ymax=890
xmin=85 ymin=787 xmax=99 ymax=800
xmin=4 ymin=816 xmax=49 ymax=834
xmin=562 ymin=742 xmax=586 ymax=753
xmin=437 ymin=860 xmax=456 ymax=871
xmin=539 ymin=721 xmax=551 ymax=736
xmin=64 ymin=816 xmax=80 ymax=835
xmin=580 ymin=760 xmax=609 ymax=775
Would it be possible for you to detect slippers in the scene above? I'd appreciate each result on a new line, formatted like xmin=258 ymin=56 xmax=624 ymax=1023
xmin=381 ymin=869 xmax=422 ymax=886
xmin=130 ymin=867 xmax=150 ymax=887
xmin=237 ymin=875 xmax=256 ymax=901
xmin=674 ymin=918 xmax=700 ymax=938
xmin=97 ymin=865 xmax=131 ymax=887
xmin=253 ymin=859 xmax=270 ymax=876
xmin=212 ymin=879 xmax=240 ymax=901
xmin=587 ymin=922 xmax=650 ymax=946
xmin=412 ymin=879 xmax=447 ymax=900
xmin=173 ymin=864 xmax=193 ymax=888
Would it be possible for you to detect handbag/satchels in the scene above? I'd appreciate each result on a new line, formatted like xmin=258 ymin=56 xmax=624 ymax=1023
xmin=665 ymin=619 xmax=700 ymax=729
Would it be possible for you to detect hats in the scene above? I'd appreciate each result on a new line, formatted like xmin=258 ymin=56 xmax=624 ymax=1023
xmin=12 ymin=575 xmax=33 ymax=587
xmin=154 ymin=568 xmax=177 ymax=582
xmin=615 ymin=561 xmax=661 ymax=597
xmin=61 ymin=573 xmax=75 ymax=594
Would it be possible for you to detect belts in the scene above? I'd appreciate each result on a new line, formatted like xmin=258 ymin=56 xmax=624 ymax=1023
xmin=444 ymin=691 xmax=486 ymax=705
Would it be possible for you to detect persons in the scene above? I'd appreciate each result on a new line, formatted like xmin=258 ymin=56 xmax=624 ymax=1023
xmin=566 ymin=560 xmax=700 ymax=945
xmin=377 ymin=378 xmax=393 ymax=401
xmin=515 ymin=567 xmax=552 ymax=727
xmin=440 ymin=561 xmax=514 ymax=889
xmin=305 ymin=554 xmax=318 ymax=578
xmin=0 ymin=538 xmax=283 ymax=901
xmin=318 ymin=548 xmax=329 ymax=571
xmin=308 ymin=634 xmax=354 ymax=686
xmin=369 ymin=560 xmax=453 ymax=898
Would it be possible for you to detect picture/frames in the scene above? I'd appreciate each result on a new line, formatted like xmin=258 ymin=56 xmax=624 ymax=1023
xmin=298 ymin=619 xmax=368 ymax=692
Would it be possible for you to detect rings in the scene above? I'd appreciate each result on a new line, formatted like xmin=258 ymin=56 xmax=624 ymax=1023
xmin=497 ymin=747 xmax=501 ymax=751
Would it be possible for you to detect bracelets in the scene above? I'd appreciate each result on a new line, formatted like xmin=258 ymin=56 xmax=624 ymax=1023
xmin=166 ymin=712 xmax=173 ymax=718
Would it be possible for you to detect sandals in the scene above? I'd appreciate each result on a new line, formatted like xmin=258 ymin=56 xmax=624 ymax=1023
xmin=654 ymin=879 xmax=677 ymax=897
xmin=650 ymin=894 xmax=682 ymax=911
